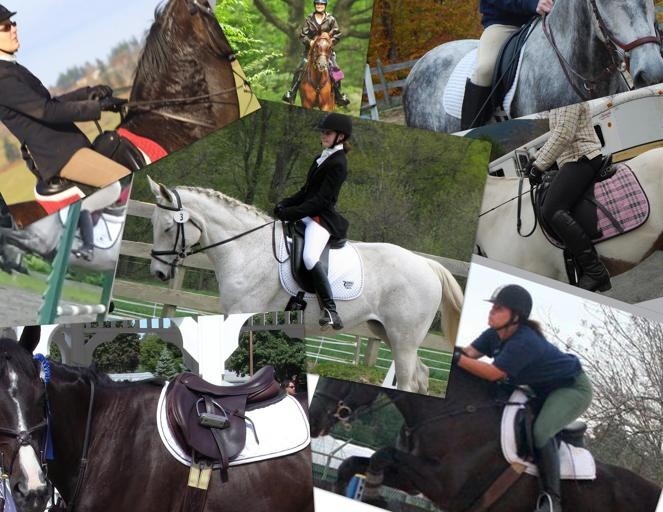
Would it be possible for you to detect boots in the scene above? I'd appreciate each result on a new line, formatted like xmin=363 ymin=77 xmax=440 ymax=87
xmin=533 ymin=437 xmax=561 ymax=512
xmin=548 ymin=209 xmax=611 ymax=293
xmin=71 ymin=209 xmax=93 ymax=261
xmin=461 ymin=78 xmax=492 ymax=131
xmin=283 ymin=70 xmax=304 ymax=103
xmin=306 ymin=261 xmax=344 ymax=330
xmin=333 ymin=79 xmax=350 ymax=106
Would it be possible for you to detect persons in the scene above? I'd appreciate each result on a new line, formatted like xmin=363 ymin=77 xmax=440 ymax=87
xmin=460 ymin=0 xmax=554 ymax=133
xmin=71 ymin=180 xmax=122 ymax=262
xmin=525 ymin=102 xmax=612 ymax=293
xmin=451 ymin=285 xmax=593 ymax=511
xmin=0 ymin=4 xmax=132 ymax=188
xmin=283 ymin=0 xmax=350 ymax=107
xmin=273 ymin=112 xmax=352 ymax=329
xmin=280 ymin=380 xmax=295 ymax=396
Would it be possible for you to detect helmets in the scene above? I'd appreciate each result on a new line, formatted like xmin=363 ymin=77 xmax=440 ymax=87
xmin=0 ymin=5 xmax=17 ymax=24
xmin=481 ymin=284 xmax=532 ymax=321
xmin=314 ymin=0 xmax=327 ymax=4
xmin=318 ymin=113 xmax=352 ymax=137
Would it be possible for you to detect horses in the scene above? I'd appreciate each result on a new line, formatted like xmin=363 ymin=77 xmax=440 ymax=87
xmin=476 ymin=146 xmax=663 ymax=287
xmin=0 ymin=326 xmax=314 ymax=512
xmin=402 ymin=0 xmax=662 ymax=137
xmin=298 ymin=28 xmax=337 ymax=112
xmin=146 ymin=174 xmax=463 ymax=395
xmin=0 ymin=1 xmax=240 ymax=231
xmin=310 ymin=345 xmax=661 ymax=512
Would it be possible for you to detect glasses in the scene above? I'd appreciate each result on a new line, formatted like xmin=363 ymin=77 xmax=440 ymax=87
xmin=290 ymin=386 xmax=296 ymax=388
xmin=1 ymin=22 xmax=16 ymax=32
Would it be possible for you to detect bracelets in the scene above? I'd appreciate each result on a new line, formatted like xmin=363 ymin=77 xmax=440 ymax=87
xmin=452 ymin=350 xmax=460 ymax=365
xmin=454 ymin=346 xmax=463 ymax=352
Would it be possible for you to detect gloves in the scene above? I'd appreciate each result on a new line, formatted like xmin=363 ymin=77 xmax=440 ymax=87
xmin=274 ymin=202 xmax=289 ymax=222
xmin=524 ymin=156 xmax=536 ymax=175
xmin=87 ymin=85 xmax=113 ymax=99
xmin=451 ymin=346 xmax=461 ymax=365
xmin=98 ymin=95 xmax=128 ymax=113
xmin=528 ymin=166 xmax=543 ymax=186
